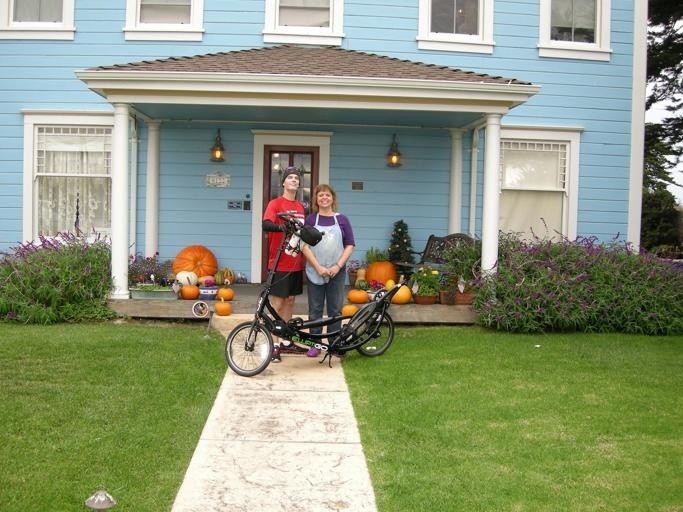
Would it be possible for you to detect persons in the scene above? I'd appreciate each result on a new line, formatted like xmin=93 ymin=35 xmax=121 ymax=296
xmin=259 ymin=166 xmax=307 ymax=364
xmin=297 ymin=181 xmax=356 ymax=361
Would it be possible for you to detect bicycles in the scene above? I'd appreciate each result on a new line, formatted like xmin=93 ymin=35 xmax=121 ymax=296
xmin=225 ymin=212 xmax=414 ymax=377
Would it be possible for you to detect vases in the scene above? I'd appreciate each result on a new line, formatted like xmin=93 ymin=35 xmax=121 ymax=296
xmin=136 ymin=283 xmax=160 ymax=288
xmin=366 ymin=289 xmax=389 ymax=303
xmin=349 ymin=272 xmax=357 ymax=286
xmin=198 ymin=287 xmax=218 ymax=301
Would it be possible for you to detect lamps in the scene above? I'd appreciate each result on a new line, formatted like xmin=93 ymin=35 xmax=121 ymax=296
xmin=209 ymin=128 xmax=225 ymax=162
xmin=387 ymin=133 xmax=402 ymax=167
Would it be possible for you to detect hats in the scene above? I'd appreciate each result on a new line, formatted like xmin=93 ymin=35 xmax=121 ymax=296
xmin=282 ymin=166 xmax=301 ymax=187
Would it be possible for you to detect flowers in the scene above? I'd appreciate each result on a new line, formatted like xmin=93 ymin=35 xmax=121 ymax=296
xmin=135 ymin=252 xmax=163 ymax=283
xmin=370 ymin=280 xmax=385 ymax=291
xmin=205 ymin=280 xmax=215 ymax=287
xmin=347 ymin=260 xmax=367 ymax=273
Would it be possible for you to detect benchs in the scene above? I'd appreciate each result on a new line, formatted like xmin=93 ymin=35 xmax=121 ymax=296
xmin=388 ymin=233 xmax=482 ymax=280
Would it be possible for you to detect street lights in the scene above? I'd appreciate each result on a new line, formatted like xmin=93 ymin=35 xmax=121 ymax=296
xmin=273 ymin=153 xmax=282 ymax=196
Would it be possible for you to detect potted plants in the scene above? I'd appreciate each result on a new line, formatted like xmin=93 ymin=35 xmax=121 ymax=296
xmin=438 ymin=242 xmax=473 ymax=304
xmin=411 ymin=268 xmax=438 ymax=305
xmin=128 ymin=285 xmax=179 ymax=300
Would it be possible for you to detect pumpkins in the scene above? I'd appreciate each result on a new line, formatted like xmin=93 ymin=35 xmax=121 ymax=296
xmin=217 ymin=282 xmax=234 ymax=301
xmin=355 ymin=264 xmax=366 ymax=288
xmin=342 ymin=303 xmax=358 ymax=317
xmin=390 ymin=284 xmax=411 ymax=304
xmin=367 ymin=261 xmax=397 ymax=286
xmin=180 ymin=277 xmax=200 ymax=300
xmin=347 ymin=288 xmax=369 ymax=304
xmin=215 ymin=269 xmax=223 ymax=285
xmin=214 ymin=297 xmax=232 ymax=316
xmin=173 ymin=245 xmax=218 ymax=278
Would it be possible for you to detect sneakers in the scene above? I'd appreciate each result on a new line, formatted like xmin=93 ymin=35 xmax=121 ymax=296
xmin=271 ymin=345 xmax=281 ymax=363
xmin=306 ymin=347 xmax=321 ymax=357
xmin=279 ymin=341 xmax=308 ymax=354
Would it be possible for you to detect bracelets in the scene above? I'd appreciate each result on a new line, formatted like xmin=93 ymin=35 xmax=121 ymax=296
xmin=335 ymin=262 xmax=342 ymax=269
